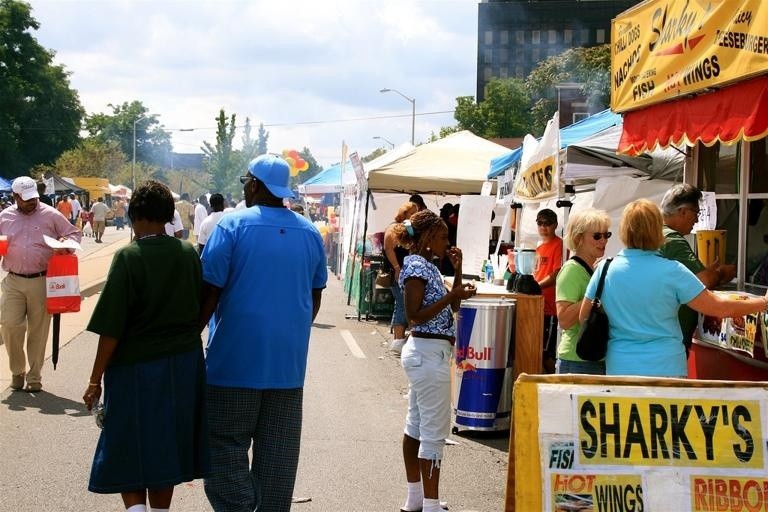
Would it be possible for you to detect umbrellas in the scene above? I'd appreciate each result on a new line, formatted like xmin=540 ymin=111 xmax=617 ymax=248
xmin=46 ymin=178 xmax=83 ymax=195
xmin=51 ymin=313 xmax=60 ymax=370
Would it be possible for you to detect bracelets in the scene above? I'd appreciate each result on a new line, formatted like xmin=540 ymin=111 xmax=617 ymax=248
xmin=759 ymin=295 xmax=768 ymax=314
xmin=87 ymin=383 xmax=102 ymax=387
xmin=394 ymin=264 xmax=398 ymax=269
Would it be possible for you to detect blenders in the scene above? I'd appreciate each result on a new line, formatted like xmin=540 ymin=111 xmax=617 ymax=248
xmin=506 ymin=247 xmax=543 ymax=297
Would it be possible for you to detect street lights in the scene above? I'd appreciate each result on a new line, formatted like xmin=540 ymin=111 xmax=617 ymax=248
xmin=380 ymin=87 xmax=415 ymax=145
xmin=371 ymin=135 xmax=394 ymax=148
xmin=132 ymin=112 xmax=160 ymax=193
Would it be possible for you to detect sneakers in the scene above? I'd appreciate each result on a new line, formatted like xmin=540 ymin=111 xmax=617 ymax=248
xmin=26 ymin=384 xmax=43 ymax=393
xmin=10 ymin=373 xmax=25 ymax=390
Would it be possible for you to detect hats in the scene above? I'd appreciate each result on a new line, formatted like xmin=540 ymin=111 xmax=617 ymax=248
xmin=248 ymin=153 xmax=297 ymax=199
xmin=71 ymin=192 xmax=76 ymax=196
xmin=11 ymin=176 xmax=40 ymax=201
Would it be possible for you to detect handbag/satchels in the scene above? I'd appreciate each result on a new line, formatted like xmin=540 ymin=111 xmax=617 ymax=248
xmin=375 ymin=253 xmax=392 ymax=288
xmin=576 ymin=257 xmax=609 ymax=362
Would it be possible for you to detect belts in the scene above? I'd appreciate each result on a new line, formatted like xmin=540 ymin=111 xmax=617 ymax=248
xmin=9 ymin=270 xmax=47 ymax=279
xmin=411 ymin=330 xmax=456 ymax=346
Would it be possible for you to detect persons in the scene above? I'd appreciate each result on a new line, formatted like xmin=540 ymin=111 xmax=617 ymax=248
xmin=87 ymin=198 xmax=110 ymax=243
xmin=83 ymin=183 xmax=211 ymax=512
xmin=221 ymin=190 xmax=249 ymax=216
xmin=194 ymin=194 xmax=209 ymax=237
xmin=305 ymin=200 xmax=323 ymax=223
xmin=650 ymin=184 xmax=736 ymax=362
xmin=394 ymin=210 xmax=477 ymax=512
xmin=113 ymin=196 xmax=125 ymax=229
xmin=578 ymin=201 xmax=768 ymax=377
xmin=69 ymin=192 xmax=81 ymax=225
xmin=173 ymin=192 xmax=194 ymax=238
xmin=55 ymin=195 xmax=73 ymax=224
xmin=198 ymin=193 xmax=225 ymax=252
xmin=439 ymin=204 xmax=459 ymax=271
xmin=0 ymin=193 xmax=15 ymax=212
xmin=383 ymin=203 xmax=417 ymax=354
xmin=35 ymin=180 xmax=52 ymax=206
xmin=0 ymin=177 xmax=84 ymax=392
xmin=554 ymin=205 xmax=611 ymax=375
xmin=198 ymin=154 xmax=326 ymax=512
xmin=534 ymin=210 xmax=563 ymax=373
xmin=165 ymin=209 xmax=183 ymax=239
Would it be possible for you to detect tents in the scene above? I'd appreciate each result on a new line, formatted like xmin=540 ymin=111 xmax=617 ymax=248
xmin=298 ymin=159 xmax=342 ymax=194
xmin=489 ymin=109 xmax=651 ymax=204
xmin=347 ymin=130 xmax=514 ymax=322
xmin=340 ymin=143 xmax=417 ymax=304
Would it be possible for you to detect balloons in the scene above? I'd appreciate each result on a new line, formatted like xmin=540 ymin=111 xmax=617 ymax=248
xmin=281 ymin=150 xmax=310 ymax=176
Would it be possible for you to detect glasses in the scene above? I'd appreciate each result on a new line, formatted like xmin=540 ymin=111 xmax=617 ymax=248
xmin=537 ymin=221 xmax=554 ymax=227
xmin=691 ymin=209 xmax=702 ymax=218
xmin=240 ymin=176 xmax=256 ymax=184
xmin=593 ymin=231 xmax=612 ymax=241
